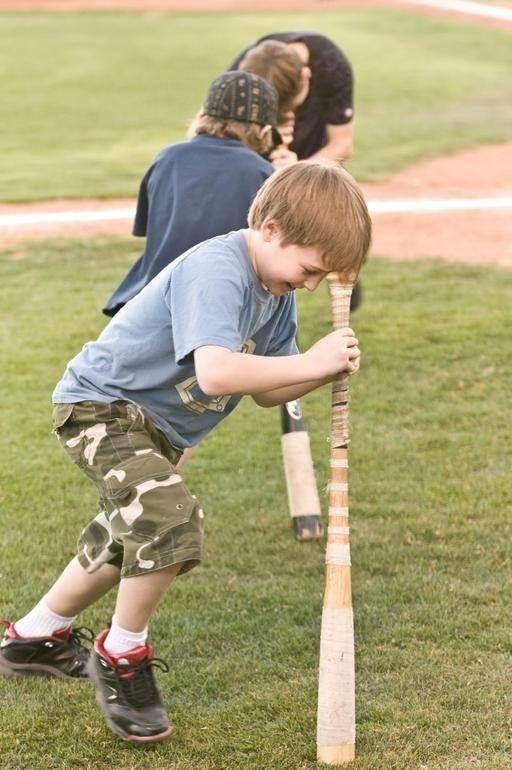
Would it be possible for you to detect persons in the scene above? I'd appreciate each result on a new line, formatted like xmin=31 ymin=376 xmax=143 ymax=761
xmin=0 ymin=157 xmax=374 ymax=745
xmin=98 ymin=67 xmax=285 ymax=321
xmin=219 ymin=31 xmax=367 ymax=313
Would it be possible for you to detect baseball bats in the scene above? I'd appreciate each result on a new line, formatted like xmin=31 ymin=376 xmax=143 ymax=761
xmin=315 ymin=267 xmax=357 ymax=766
xmin=279 ymin=399 xmax=325 ymax=543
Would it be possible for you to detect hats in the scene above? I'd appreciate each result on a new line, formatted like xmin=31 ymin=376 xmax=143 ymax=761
xmin=201 ymin=69 xmax=283 ymax=148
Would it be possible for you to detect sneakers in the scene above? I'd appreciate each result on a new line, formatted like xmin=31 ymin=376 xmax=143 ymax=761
xmin=86 ymin=622 xmax=172 ymax=744
xmin=0 ymin=621 xmax=97 ymax=682
